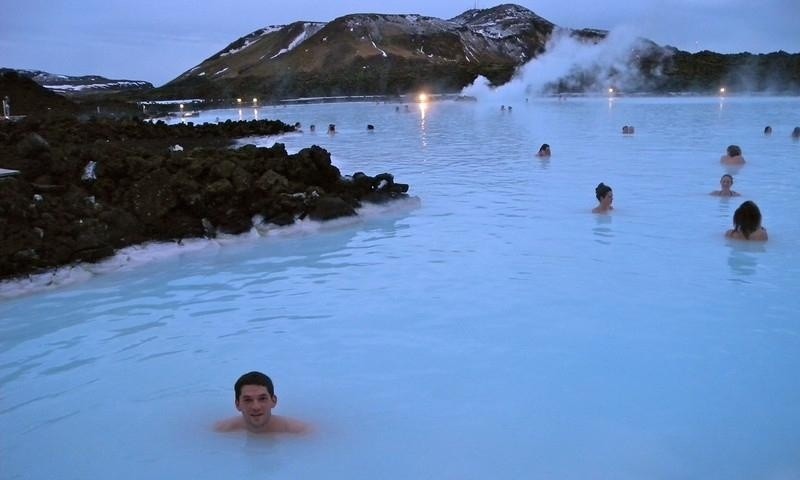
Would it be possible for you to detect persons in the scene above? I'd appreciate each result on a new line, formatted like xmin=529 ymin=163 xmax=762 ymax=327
xmin=764 ymin=127 xmax=772 ymax=133
xmin=214 ymin=372 xmax=312 ymax=434
xmin=535 ymin=143 xmax=552 ymax=157
xmin=593 ymin=183 xmax=616 ymax=213
xmin=792 ymin=127 xmax=800 ymax=136
xmin=500 ymin=105 xmax=512 ymax=111
xmin=622 ymin=126 xmax=634 ymax=134
xmin=368 ymin=125 xmax=373 ymax=129
xmin=721 ymin=145 xmax=745 ymax=164
xmin=710 ymin=175 xmax=741 ymax=196
xmin=395 ymin=105 xmax=410 ymax=113
xmin=294 ymin=122 xmax=337 ymax=134
xmin=725 ymin=201 xmax=768 ymax=240
